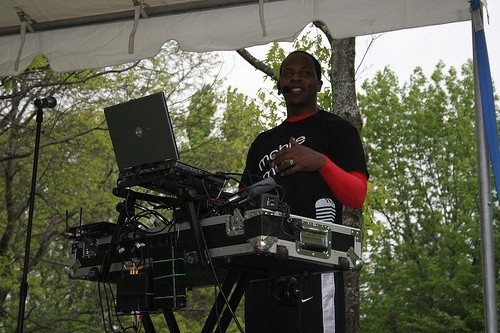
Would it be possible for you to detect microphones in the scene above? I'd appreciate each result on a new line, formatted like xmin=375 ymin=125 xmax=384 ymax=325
xmin=34 ymin=97 xmax=57 ymax=108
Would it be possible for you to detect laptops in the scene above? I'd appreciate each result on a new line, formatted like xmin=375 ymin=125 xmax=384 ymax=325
xmin=103 ymin=92 xmax=227 ymax=196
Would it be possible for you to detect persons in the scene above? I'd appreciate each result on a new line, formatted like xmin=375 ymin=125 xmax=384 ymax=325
xmin=238 ymin=51 xmax=369 ymax=333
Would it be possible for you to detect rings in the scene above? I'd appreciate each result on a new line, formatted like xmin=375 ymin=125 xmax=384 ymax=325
xmin=290 ymin=159 xmax=293 ymax=166
